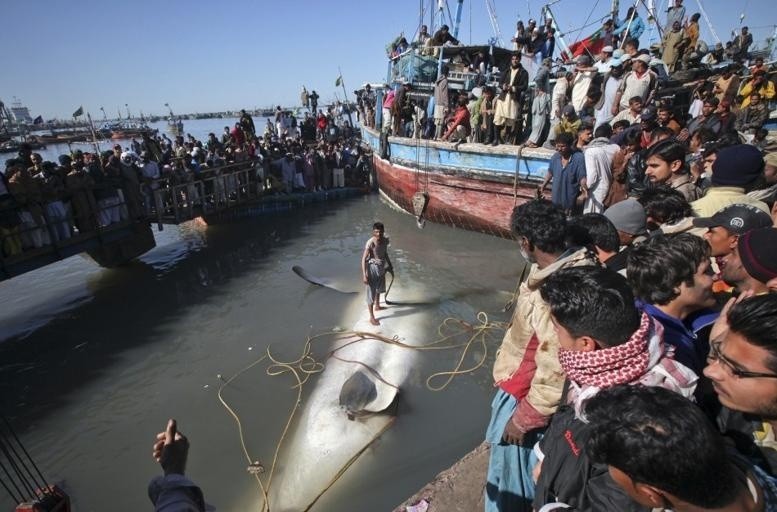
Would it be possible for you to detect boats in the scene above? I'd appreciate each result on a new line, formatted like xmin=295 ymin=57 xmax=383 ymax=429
xmin=347 ymin=1 xmax=775 ymax=245
xmin=0 ymin=102 xmax=184 ymax=152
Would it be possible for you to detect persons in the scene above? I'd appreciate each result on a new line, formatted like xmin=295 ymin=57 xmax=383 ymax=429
xmin=361 ymin=222 xmax=393 ymax=324
xmin=148 ymin=418 xmax=207 ymax=510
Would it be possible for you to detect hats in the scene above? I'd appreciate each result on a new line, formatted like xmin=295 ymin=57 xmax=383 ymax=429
xmin=631 ymin=53 xmax=652 ymax=65
xmin=4 ymin=158 xmax=18 ymax=166
xmin=737 ymin=226 xmax=777 ymax=284
xmin=710 ymin=144 xmax=765 ymax=186
xmin=601 ymin=45 xmax=631 ymax=67
xmin=112 ymin=143 xmax=121 ymax=150
xmin=576 ymin=54 xmax=592 ymax=66
xmin=40 ymin=161 xmax=57 ymax=170
xmin=692 ymin=202 xmax=774 ymax=237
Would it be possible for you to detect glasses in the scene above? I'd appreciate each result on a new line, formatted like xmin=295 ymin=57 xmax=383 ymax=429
xmin=711 ymin=336 xmax=777 ymax=381
